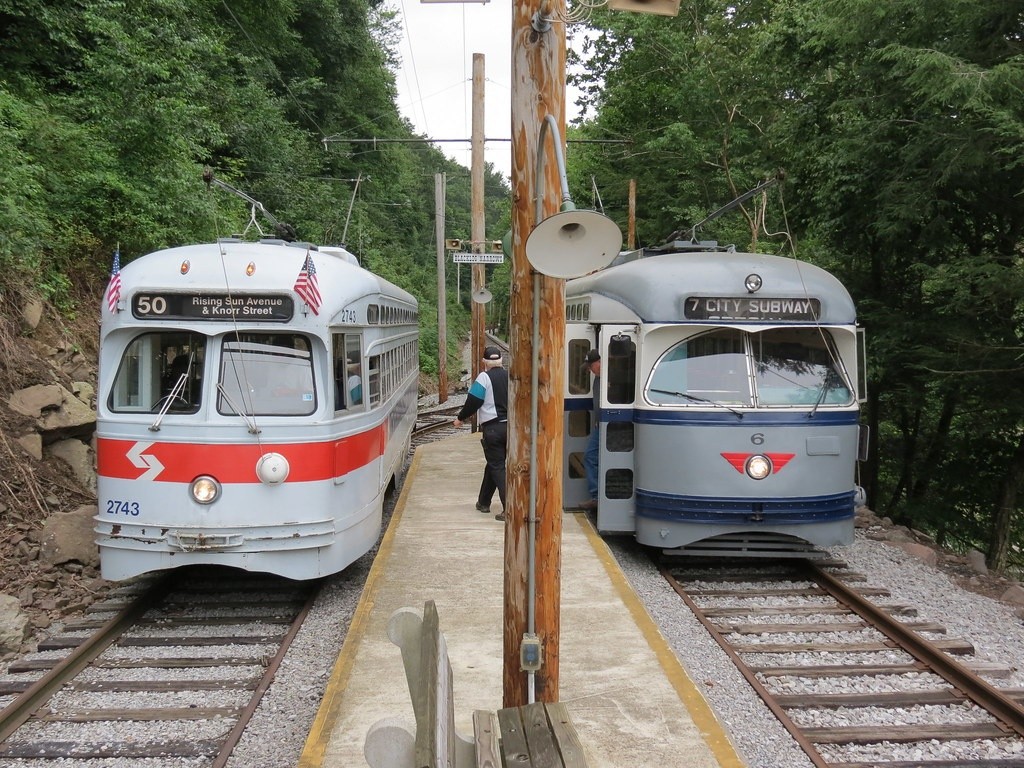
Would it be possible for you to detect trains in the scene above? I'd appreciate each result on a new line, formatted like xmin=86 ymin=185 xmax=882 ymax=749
xmin=560 ymin=168 xmax=870 ymax=559
xmin=92 ymin=198 xmax=419 ymax=580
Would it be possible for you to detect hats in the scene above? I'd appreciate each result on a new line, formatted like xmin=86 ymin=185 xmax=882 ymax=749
xmin=348 ymin=350 xmax=360 ymax=364
xmin=580 ymin=349 xmax=601 ymax=368
xmin=483 ymin=347 xmax=501 ymax=360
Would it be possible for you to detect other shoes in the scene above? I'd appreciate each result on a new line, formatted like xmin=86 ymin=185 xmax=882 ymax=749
xmin=476 ymin=502 xmax=490 ymax=512
xmin=495 ymin=511 xmax=505 ymax=521
xmin=579 ymin=498 xmax=598 ymax=508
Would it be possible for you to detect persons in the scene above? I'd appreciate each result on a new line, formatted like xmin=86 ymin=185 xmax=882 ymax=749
xmin=347 ymin=351 xmax=362 ymax=405
xmin=579 ymin=350 xmax=601 ymax=509
xmin=452 ymin=347 xmax=507 ymax=521
xmin=244 ymin=336 xmax=312 ymax=415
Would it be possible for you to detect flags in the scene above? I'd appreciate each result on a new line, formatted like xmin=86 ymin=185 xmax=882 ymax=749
xmin=294 ymin=252 xmax=323 ymax=316
xmin=105 ymin=248 xmax=121 ymax=314
